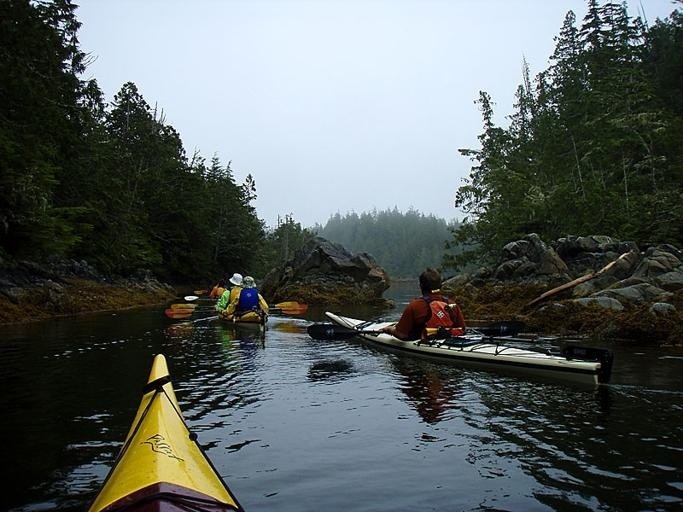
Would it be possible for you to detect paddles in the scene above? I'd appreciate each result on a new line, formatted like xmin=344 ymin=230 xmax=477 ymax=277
xmin=171 ymin=301 xmax=299 ymax=309
xmin=184 ymin=296 xmax=221 ymax=302
xmin=307 ymin=321 xmax=524 ymax=340
xmin=194 ymin=290 xmax=208 ymax=295
xmin=165 ymin=304 xmax=308 ymax=318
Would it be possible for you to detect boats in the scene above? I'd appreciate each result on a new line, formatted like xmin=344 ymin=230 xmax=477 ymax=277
xmin=323 ymin=309 xmax=614 ymax=388
xmin=215 ymin=304 xmax=266 ymax=324
xmin=87 ymin=351 xmax=248 ymax=512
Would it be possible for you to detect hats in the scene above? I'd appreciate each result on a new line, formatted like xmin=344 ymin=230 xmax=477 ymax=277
xmin=229 ymin=273 xmax=243 ymax=286
xmin=240 ymin=276 xmax=257 ymax=289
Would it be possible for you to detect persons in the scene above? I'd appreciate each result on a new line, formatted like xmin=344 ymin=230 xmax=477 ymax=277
xmin=211 ymin=273 xmax=269 ymax=319
xmin=380 ymin=268 xmax=466 ymax=341
